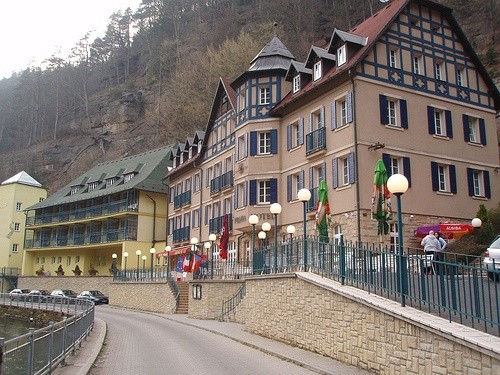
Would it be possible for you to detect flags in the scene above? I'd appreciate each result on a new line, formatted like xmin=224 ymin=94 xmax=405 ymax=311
xmin=176 ymin=253 xmax=203 ymax=273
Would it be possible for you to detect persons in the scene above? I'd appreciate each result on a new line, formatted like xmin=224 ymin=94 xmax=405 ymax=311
xmin=421 ymin=230 xmax=441 ymax=274
xmin=434 ymin=231 xmax=447 ymax=252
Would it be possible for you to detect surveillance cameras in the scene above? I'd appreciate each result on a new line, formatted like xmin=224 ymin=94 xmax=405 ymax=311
xmin=410 ymin=215 xmax=414 ymax=218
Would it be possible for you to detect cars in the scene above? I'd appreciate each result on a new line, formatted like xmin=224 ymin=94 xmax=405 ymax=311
xmin=483 ymin=235 xmax=500 ymax=281
xmin=9 ymin=289 xmax=31 ymax=302
xmin=27 ymin=289 xmax=52 ymax=303
xmin=50 ymin=289 xmax=78 ymax=304
xmin=76 ymin=291 xmax=109 ymax=305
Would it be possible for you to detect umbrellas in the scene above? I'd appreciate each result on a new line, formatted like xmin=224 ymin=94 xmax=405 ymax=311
xmin=370 ymin=159 xmax=393 ymax=268
xmin=314 ymin=180 xmax=331 ymax=246
xmin=414 ymin=221 xmax=474 ymax=240
xmin=219 ymin=214 xmax=228 ymax=260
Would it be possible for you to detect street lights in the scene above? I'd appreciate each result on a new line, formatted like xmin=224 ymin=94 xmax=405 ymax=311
xmin=262 ymin=222 xmax=271 ymax=255
xmin=204 ymin=242 xmax=211 ymax=278
xmin=249 ymin=214 xmax=259 ymax=274
xmin=191 ymin=237 xmax=198 ymax=280
xmin=150 ymin=248 xmax=156 ymax=281
xmin=166 ymin=246 xmax=171 ymax=278
xmin=258 ymin=230 xmax=266 ymax=266
xmin=136 ymin=249 xmax=141 ymax=281
xmin=142 ymin=256 xmax=147 ymax=273
xmin=472 ymin=218 xmax=483 ymax=281
xmin=287 ymin=225 xmax=295 ymax=271
xmin=112 ymin=253 xmax=117 ymax=280
xmin=298 ymin=188 xmax=312 ymax=270
xmin=386 ymin=173 xmax=409 ymax=307
xmin=270 ymin=203 xmax=282 ymax=273
xmin=123 ymin=251 xmax=129 ymax=281
xmin=208 ymin=233 xmax=216 ymax=279
